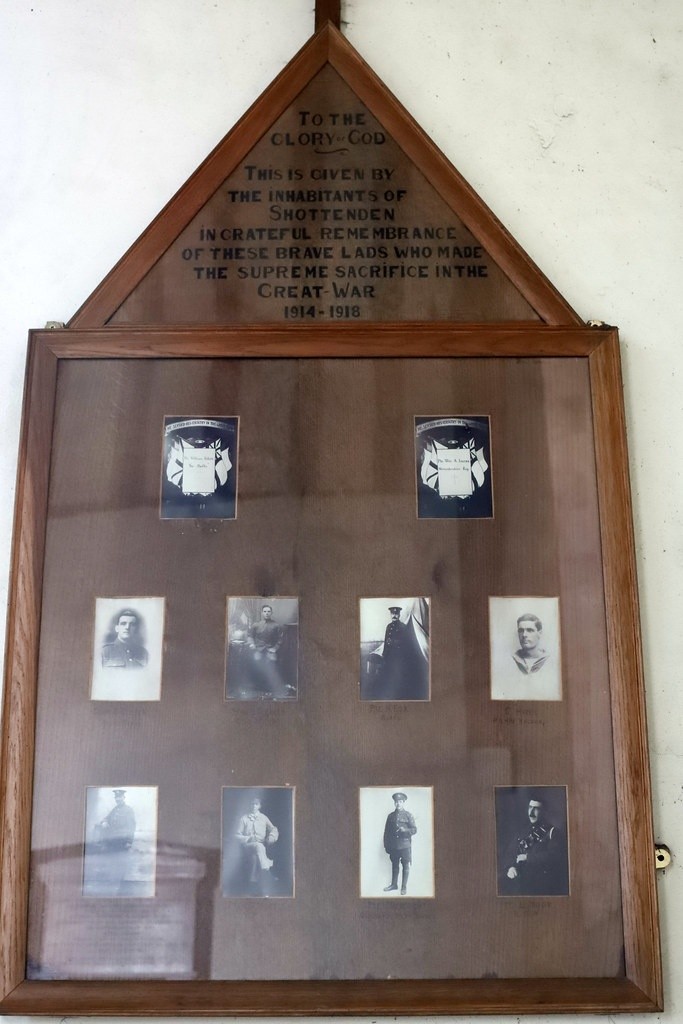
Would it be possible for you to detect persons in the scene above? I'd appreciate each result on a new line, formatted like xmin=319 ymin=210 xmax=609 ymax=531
xmin=101 ymin=611 xmax=149 ymax=669
xmin=247 ymin=604 xmax=285 ymax=692
xmin=510 ymin=613 xmax=552 ymax=676
xmin=236 ymin=797 xmax=282 ymax=897
xmin=502 ymin=795 xmax=562 ymax=895
xmin=383 ymin=792 xmax=417 ymax=895
xmin=382 ymin=606 xmax=411 ymax=700
xmin=101 ymin=788 xmax=137 ymax=889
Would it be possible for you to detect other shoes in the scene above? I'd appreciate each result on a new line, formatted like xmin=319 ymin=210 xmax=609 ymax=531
xmin=384 ymin=884 xmax=398 ymax=891
xmin=400 ymin=885 xmax=406 ymax=894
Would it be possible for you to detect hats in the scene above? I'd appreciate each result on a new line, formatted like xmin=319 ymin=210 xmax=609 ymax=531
xmin=113 ymin=790 xmax=126 ymax=795
xmin=388 ymin=607 xmax=402 ymax=613
xmin=392 ymin=792 xmax=407 ymax=801
